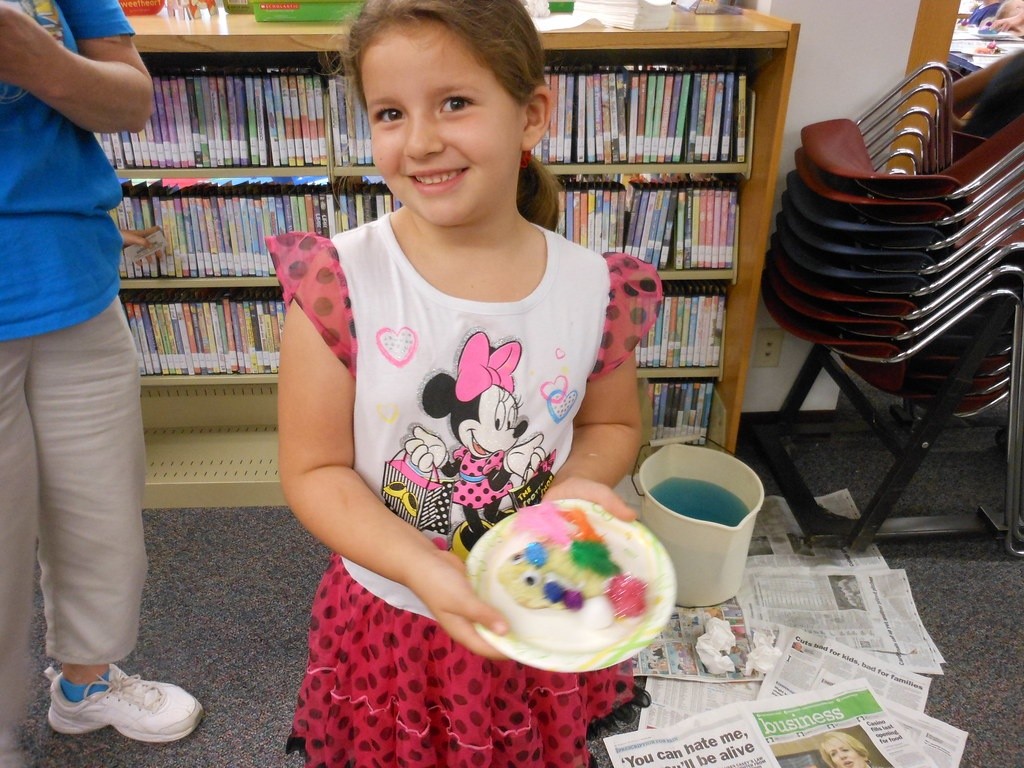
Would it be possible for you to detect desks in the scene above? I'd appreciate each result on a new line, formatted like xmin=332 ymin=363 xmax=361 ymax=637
xmin=949 ymin=18 xmax=1024 ymax=79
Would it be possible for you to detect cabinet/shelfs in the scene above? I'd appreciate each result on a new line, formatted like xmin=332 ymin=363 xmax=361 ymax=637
xmin=86 ymin=0 xmax=803 ymax=512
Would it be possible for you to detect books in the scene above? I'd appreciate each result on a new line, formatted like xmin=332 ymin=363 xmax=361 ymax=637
xmin=528 ymin=58 xmax=749 ymax=444
xmin=94 ymin=65 xmax=403 ymax=378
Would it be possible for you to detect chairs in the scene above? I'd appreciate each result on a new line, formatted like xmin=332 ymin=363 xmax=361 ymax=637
xmin=751 ymin=60 xmax=1024 ymax=562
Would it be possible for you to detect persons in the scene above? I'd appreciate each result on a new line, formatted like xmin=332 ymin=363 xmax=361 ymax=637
xmin=266 ymin=1 xmax=663 ymax=768
xmin=969 ymin=0 xmax=1024 ymax=40
xmin=119 ymin=226 xmax=162 ymax=266
xmin=0 ymin=0 xmax=203 ymax=768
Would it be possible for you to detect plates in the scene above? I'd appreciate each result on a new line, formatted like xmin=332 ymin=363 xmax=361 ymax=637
xmin=467 ymin=498 xmax=677 ymax=672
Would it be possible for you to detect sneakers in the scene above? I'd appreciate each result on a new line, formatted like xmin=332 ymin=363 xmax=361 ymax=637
xmin=44 ymin=664 xmax=203 ymax=742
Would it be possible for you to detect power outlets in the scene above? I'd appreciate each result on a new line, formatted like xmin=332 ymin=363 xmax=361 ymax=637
xmin=752 ymin=327 xmax=786 ymax=368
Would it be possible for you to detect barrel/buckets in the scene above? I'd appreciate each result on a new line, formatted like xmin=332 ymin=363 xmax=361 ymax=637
xmin=640 ymin=443 xmax=764 ymax=608
